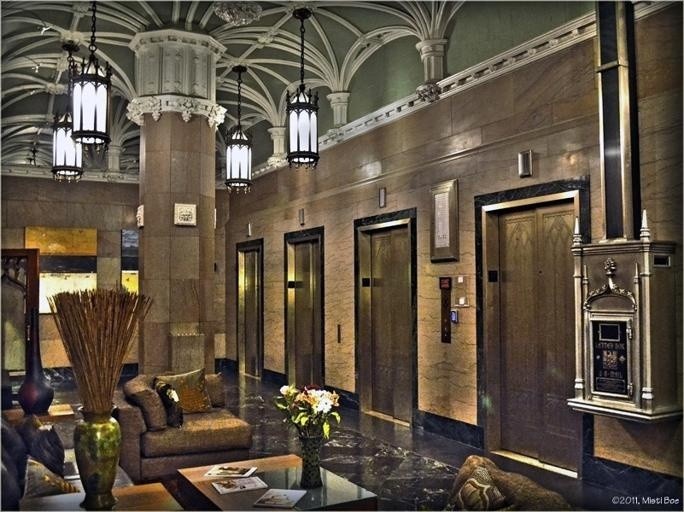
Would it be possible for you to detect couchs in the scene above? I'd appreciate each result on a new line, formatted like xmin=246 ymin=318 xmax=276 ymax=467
xmin=446 ymin=454 xmax=572 ymax=511
xmin=112 ymin=373 xmax=252 ymax=483
xmin=0 ymin=412 xmax=134 ymax=512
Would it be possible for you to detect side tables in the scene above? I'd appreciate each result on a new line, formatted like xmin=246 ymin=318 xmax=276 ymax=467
xmin=20 ymin=482 xmax=183 ymax=511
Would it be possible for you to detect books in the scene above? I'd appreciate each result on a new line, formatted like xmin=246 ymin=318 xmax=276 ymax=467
xmin=211 ymin=476 xmax=268 ymax=495
xmin=252 ymin=488 xmax=308 ymax=508
xmin=204 ymin=465 xmax=257 ymax=478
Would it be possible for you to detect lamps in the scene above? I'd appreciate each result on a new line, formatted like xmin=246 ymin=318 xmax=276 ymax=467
xmin=285 ymin=8 xmax=320 ymax=169
xmin=51 ymin=40 xmax=83 ymax=182
xmin=226 ymin=65 xmax=254 ymax=194
xmin=70 ymin=1 xmax=113 ymax=157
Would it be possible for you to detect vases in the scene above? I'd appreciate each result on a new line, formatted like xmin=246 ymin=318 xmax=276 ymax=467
xmin=15 ymin=304 xmax=57 ymax=416
xmin=70 ymin=403 xmax=126 ymax=510
xmin=298 ymin=433 xmax=324 ymax=488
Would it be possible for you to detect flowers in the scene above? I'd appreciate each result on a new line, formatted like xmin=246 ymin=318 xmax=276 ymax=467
xmin=277 ymin=384 xmax=341 ymax=440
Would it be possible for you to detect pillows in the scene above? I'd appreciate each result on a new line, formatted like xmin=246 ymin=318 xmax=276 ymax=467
xmin=153 ymin=368 xmax=213 ymax=413
xmin=18 ymin=457 xmax=81 ymax=512
xmin=31 ymin=424 xmax=65 ymax=477
xmin=441 ymin=467 xmax=504 ymax=511
xmin=123 ymin=380 xmax=167 ymax=431
xmin=152 ymin=377 xmax=184 ymax=429
